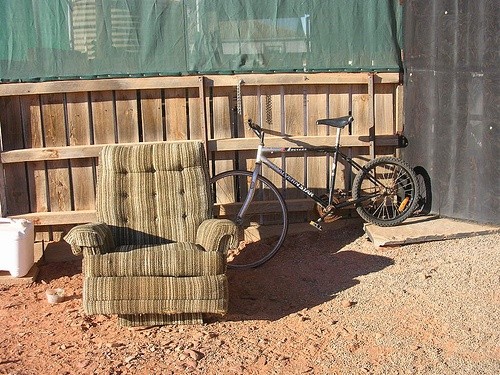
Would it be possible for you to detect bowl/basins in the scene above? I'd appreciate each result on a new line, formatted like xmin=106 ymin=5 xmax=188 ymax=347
xmin=46 ymin=288 xmax=64 ymax=303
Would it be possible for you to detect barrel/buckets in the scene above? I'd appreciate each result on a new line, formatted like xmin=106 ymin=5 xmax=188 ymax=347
xmin=0 ymin=218 xmax=34 ymax=278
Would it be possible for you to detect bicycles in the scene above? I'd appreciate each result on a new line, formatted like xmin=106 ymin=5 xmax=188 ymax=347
xmin=209 ymin=114 xmax=421 ymax=270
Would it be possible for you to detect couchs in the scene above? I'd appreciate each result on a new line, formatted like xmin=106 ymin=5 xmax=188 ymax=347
xmin=63 ymin=140 xmax=240 ymax=328
